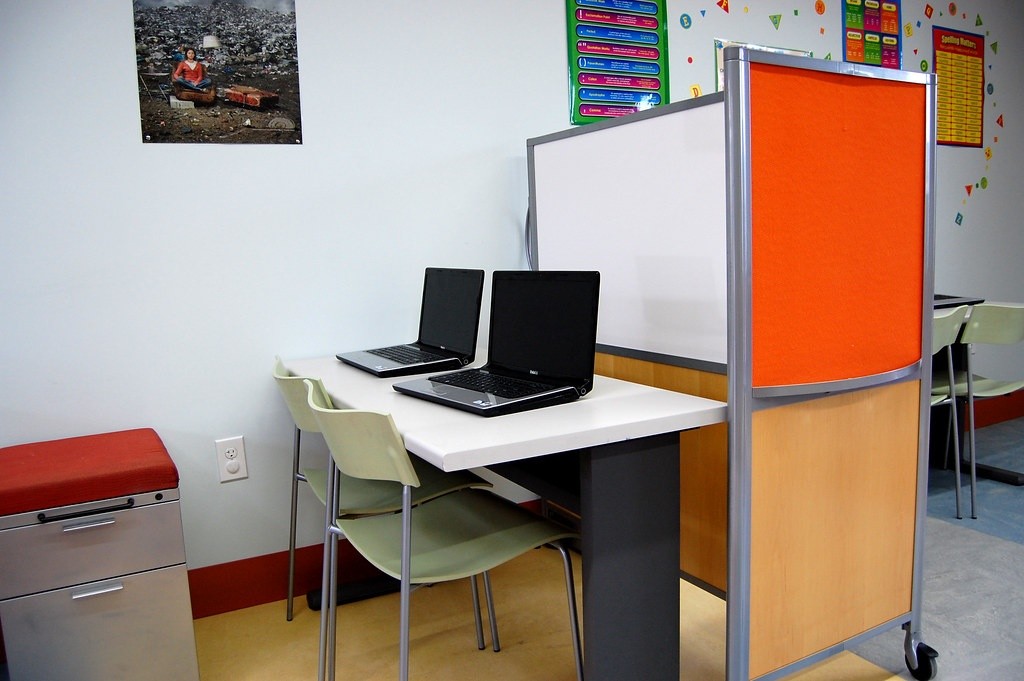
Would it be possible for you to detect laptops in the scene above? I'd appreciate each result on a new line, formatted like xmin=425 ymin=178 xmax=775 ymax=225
xmin=335 ymin=267 xmax=485 ymax=378
xmin=393 ymin=270 xmax=602 ymax=418
xmin=934 ymin=294 xmax=985 ymax=310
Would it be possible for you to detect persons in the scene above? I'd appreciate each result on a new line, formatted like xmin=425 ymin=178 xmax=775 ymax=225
xmin=173 ymin=48 xmax=213 ymax=93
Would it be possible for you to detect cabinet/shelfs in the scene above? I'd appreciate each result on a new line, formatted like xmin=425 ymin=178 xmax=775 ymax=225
xmin=0 ymin=427 xmax=202 ymax=681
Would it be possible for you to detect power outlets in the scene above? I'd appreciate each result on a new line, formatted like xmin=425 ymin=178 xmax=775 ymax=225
xmin=216 ymin=436 xmax=248 ymax=482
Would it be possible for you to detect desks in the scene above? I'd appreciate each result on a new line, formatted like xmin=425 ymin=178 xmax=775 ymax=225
xmin=284 ymin=340 xmax=729 ymax=681
xmin=139 ymin=72 xmax=170 ymax=103
xmin=928 ymin=294 xmax=1024 ymax=489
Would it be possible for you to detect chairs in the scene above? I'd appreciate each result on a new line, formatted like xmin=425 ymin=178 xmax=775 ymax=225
xmin=931 ymin=305 xmax=969 ymax=519
xmin=273 ymin=354 xmax=486 ymax=681
xmin=301 ymin=378 xmax=584 ymax=681
xmin=926 ymin=304 xmax=1024 ymax=520
